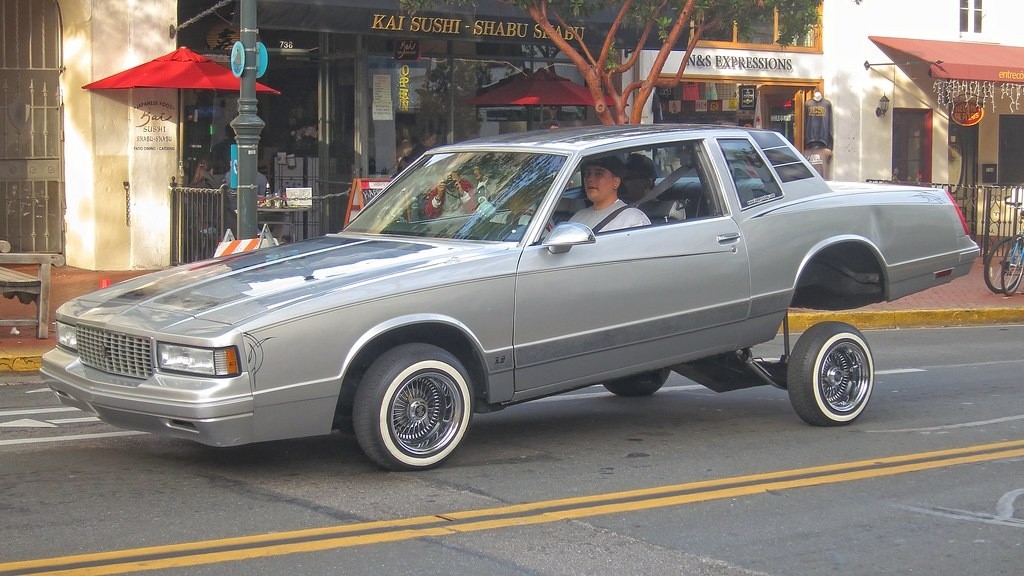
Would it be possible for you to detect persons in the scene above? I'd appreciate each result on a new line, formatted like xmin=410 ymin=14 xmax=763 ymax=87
xmin=566 ymin=156 xmax=651 ymax=233
xmin=409 ymin=128 xmax=440 ymax=160
xmin=193 ymin=162 xmax=220 ymax=192
xmin=223 ymin=166 xmax=272 ymax=212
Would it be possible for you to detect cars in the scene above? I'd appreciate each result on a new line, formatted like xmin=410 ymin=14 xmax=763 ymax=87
xmin=39 ymin=123 xmax=981 ymax=471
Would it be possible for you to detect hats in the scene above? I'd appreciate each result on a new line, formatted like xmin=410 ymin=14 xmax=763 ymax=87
xmin=622 ymin=153 xmax=657 ymax=179
xmin=588 ymin=155 xmax=624 ymax=179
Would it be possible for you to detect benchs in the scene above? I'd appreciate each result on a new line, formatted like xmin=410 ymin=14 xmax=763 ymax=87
xmin=654 ymin=182 xmax=713 ymax=217
xmin=0 ymin=239 xmax=65 ymax=338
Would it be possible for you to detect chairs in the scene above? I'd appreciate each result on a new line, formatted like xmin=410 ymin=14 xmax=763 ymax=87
xmin=616 ymin=164 xmax=668 ymax=227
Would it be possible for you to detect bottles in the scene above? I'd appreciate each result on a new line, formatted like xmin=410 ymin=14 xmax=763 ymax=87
xmin=265 ymin=181 xmax=271 ymax=207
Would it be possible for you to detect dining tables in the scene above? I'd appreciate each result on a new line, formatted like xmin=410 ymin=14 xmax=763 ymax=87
xmin=257 ymin=204 xmax=313 ymax=242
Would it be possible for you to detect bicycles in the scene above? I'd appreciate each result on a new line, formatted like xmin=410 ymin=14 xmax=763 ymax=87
xmin=984 ymin=196 xmax=1024 ymax=294
xmin=1001 ymin=234 xmax=1024 ymax=297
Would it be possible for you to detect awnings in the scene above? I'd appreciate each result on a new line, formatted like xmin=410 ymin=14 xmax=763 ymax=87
xmin=864 ymin=35 xmax=1024 ymax=85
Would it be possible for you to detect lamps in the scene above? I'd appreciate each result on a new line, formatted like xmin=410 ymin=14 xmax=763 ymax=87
xmin=875 ymin=90 xmax=890 ymax=115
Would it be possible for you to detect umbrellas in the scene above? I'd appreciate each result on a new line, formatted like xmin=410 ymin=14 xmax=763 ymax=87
xmin=80 ymin=46 xmax=283 ymax=266
xmin=465 ymin=70 xmax=631 ymax=130
